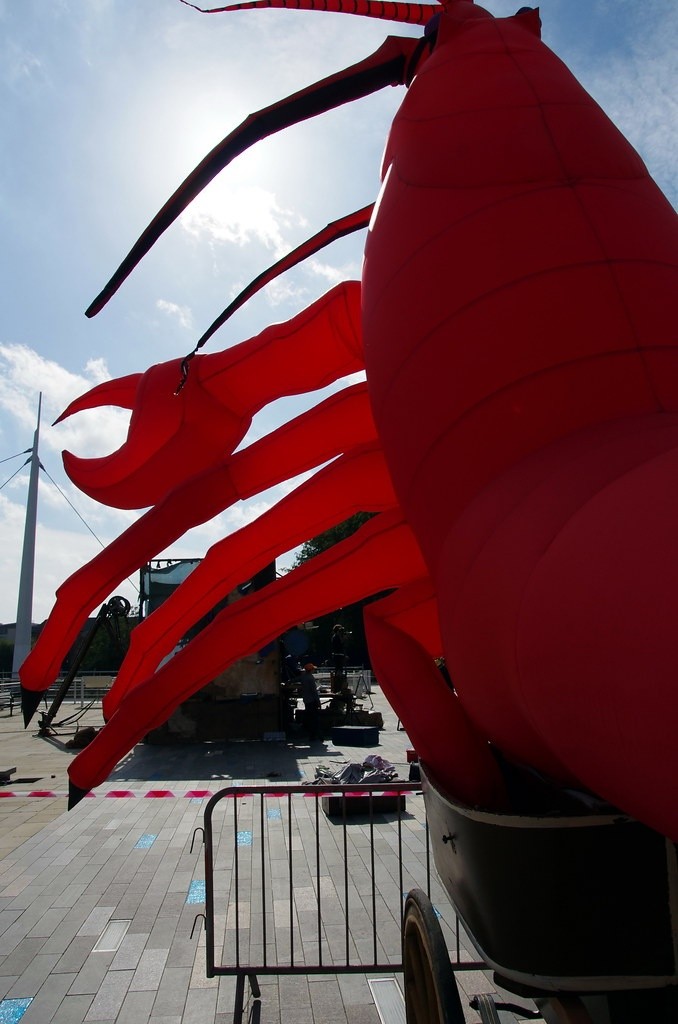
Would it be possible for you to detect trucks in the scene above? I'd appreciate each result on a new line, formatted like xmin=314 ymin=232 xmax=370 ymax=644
xmin=133 ymin=555 xmax=286 ymax=742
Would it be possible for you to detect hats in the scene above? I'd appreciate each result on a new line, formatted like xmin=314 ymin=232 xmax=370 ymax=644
xmin=305 ymin=663 xmax=317 ymax=671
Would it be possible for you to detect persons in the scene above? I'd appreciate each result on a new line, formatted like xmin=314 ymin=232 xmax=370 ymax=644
xmin=333 ymin=666 xmax=354 ymax=714
xmin=330 ymin=624 xmax=350 ymax=666
xmin=300 ymin=662 xmax=326 ymax=742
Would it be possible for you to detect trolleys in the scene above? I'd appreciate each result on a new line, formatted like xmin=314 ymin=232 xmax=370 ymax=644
xmin=398 ymin=757 xmax=678 ymax=1024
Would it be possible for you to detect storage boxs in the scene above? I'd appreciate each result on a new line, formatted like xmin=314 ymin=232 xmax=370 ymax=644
xmin=331 ymin=725 xmax=382 ymax=746
